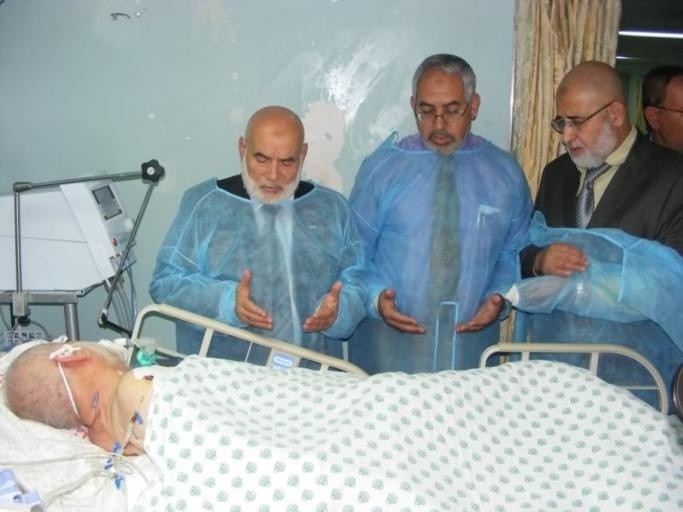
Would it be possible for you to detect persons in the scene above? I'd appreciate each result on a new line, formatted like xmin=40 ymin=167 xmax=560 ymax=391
xmin=508 ymin=61 xmax=683 ymax=412
xmin=347 ymin=52 xmax=534 ymax=378
xmin=640 ymin=64 xmax=683 ymax=153
xmin=148 ymin=105 xmax=373 ymax=373
xmin=5 ymin=337 xmax=152 ymax=456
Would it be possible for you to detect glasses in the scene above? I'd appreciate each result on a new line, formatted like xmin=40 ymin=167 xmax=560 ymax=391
xmin=413 ymin=101 xmax=469 ymax=123
xmin=551 ymin=98 xmax=614 ymax=133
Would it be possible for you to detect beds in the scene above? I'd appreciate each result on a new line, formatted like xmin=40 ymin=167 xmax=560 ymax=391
xmin=1 ymin=303 xmax=683 ymax=512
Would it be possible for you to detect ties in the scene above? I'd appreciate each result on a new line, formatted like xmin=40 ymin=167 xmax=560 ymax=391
xmin=575 ymin=163 xmax=612 ymax=231
xmin=431 ymin=156 xmax=458 ymax=311
xmin=257 ymin=205 xmax=294 ymax=343
